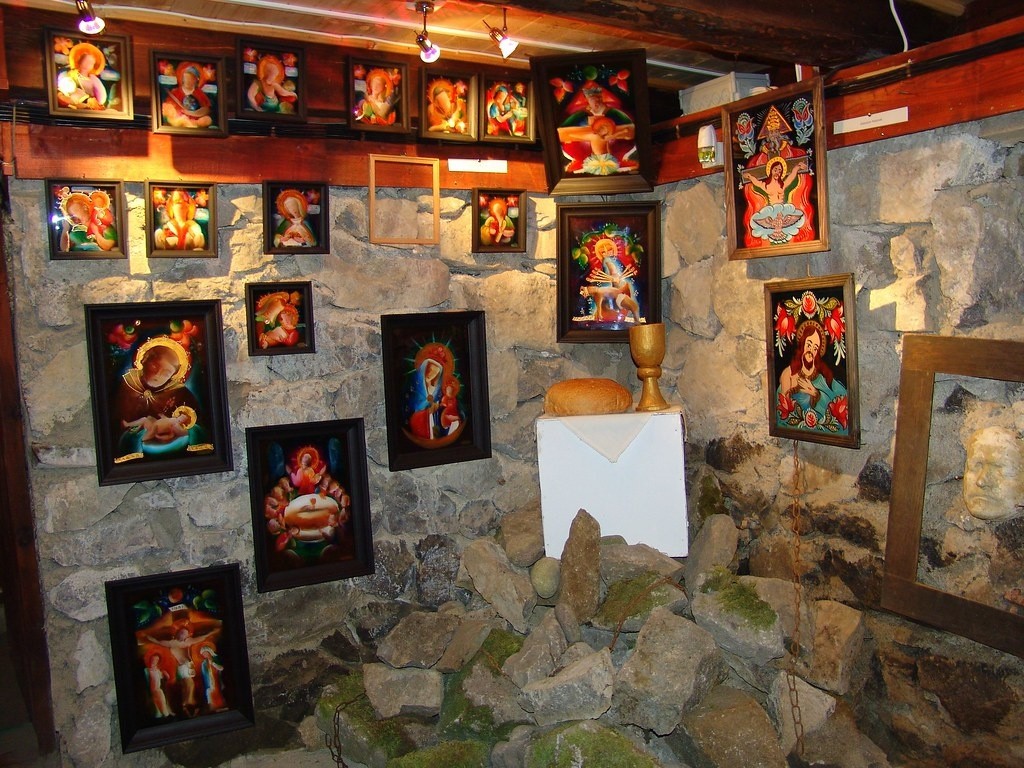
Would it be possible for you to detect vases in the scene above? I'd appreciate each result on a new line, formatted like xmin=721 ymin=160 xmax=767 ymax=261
xmin=629 ymin=323 xmax=672 ymax=412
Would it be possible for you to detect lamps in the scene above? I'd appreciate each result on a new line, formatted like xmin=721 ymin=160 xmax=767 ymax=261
xmin=413 ymin=0 xmax=441 ymax=63
xmin=75 ymin=0 xmax=106 ymax=35
xmin=696 ymin=124 xmax=724 ymax=170
xmin=481 ymin=4 xmax=520 ymax=59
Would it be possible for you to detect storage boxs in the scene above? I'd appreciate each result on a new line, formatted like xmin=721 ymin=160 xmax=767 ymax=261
xmin=534 ymin=403 xmax=690 ymax=558
xmin=679 ymin=71 xmax=770 ymax=116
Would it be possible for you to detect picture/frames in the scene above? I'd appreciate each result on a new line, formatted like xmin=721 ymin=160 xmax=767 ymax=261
xmin=526 ymin=45 xmax=655 ymax=196
xmin=149 ymin=47 xmax=231 ymax=139
xmin=369 ymin=153 xmax=440 ymax=245
xmin=346 ymin=55 xmax=412 ymax=135
xmin=262 ymin=179 xmax=329 ymax=256
xmin=764 ymin=271 xmax=860 ymax=450
xmin=380 ymin=308 xmax=494 ymax=472
xmin=144 ymin=178 xmax=219 ymax=259
xmin=45 ymin=176 xmax=127 ymax=260
xmin=244 ymin=418 xmax=376 ymax=593
xmin=555 ymin=198 xmax=662 ymax=343
xmin=244 ymin=278 xmax=315 ymax=357
xmin=83 ymin=299 xmax=235 ymax=485
xmin=418 ymin=64 xmax=479 ymax=146
xmin=721 ymin=75 xmax=829 ymax=260
xmin=104 ymin=562 xmax=255 ymax=755
xmin=235 ymin=34 xmax=309 ymax=125
xmin=42 ymin=23 xmax=135 ymax=123
xmin=479 ymin=69 xmax=536 ymax=144
xmin=472 ymin=185 xmax=529 ymax=253
xmin=877 ymin=333 xmax=1024 ymax=662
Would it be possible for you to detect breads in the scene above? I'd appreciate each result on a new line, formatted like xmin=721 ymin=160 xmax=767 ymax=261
xmin=544 ymin=377 xmax=633 ymax=416
